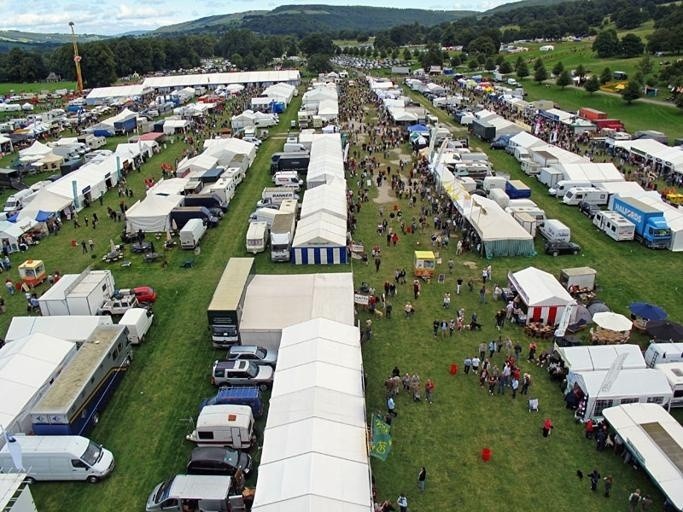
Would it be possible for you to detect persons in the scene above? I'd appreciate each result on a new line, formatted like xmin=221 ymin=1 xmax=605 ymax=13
xmin=1 ymin=83 xmax=267 ymax=316
xmin=338 ymin=77 xmax=683 ymax=512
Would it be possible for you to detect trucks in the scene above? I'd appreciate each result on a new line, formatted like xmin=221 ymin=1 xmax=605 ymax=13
xmin=114 ymin=84 xmax=243 ymax=134
xmin=573 ymin=106 xmax=669 ymax=145
xmin=489 ymin=133 xmax=557 ymax=177
xmin=29 ymin=135 xmax=111 ymax=172
xmin=169 ymin=164 xmax=244 ymax=250
xmin=242 ymin=141 xmax=310 ymax=262
xmin=99 ymin=285 xmax=157 ymax=342
xmin=289 ymin=72 xmax=340 ymax=133
xmin=0 ymin=169 xmax=65 ymax=224
xmin=422 ymin=115 xmax=494 ymax=176
xmin=225 ymin=95 xmax=284 ymax=140
xmin=205 ymin=255 xmax=255 ymax=350
xmin=29 ymin=323 xmax=134 ymax=438
xmin=549 ymin=179 xmax=672 ymax=251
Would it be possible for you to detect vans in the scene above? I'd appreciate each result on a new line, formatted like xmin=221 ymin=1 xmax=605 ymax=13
xmin=458 ymin=173 xmax=582 ymax=256
xmin=0 ymin=430 xmax=117 ymax=484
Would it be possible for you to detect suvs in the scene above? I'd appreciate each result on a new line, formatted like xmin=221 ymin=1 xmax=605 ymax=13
xmin=145 ymin=344 xmax=277 ymax=511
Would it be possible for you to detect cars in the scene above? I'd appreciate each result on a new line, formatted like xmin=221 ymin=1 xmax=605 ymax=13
xmin=0 ymin=89 xmax=115 ymax=142
xmin=614 ymin=71 xmax=627 ymax=81
xmin=403 ymin=75 xmax=471 ymax=114
xmin=501 ymin=45 xmax=528 ymax=54
xmin=539 ymin=45 xmax=555 ymax=52
xmin=477 ymin=73 xmax=524 ymax=100
